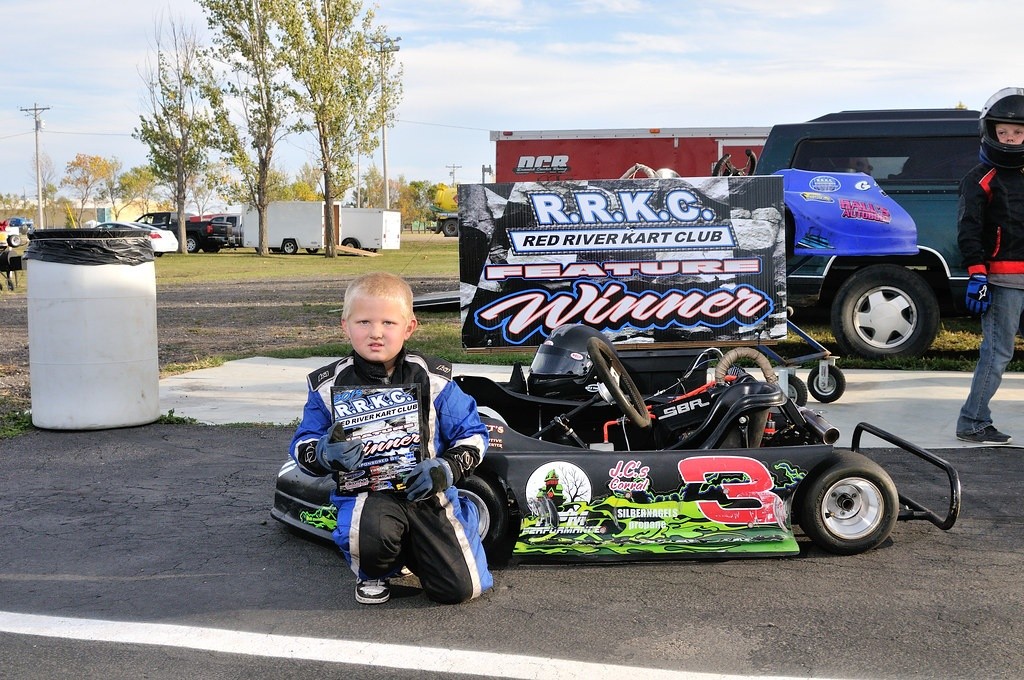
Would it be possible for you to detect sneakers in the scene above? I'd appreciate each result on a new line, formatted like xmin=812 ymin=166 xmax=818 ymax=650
xmin=354 ymin=576 xmax=391 ymax=604
xmin=393 ymin=561 xmax=413 ymax=577
xmin=956 ymin=424 xmax=1013 ymax=445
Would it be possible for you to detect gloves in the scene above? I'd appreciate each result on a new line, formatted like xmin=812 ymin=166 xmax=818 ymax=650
xmin=965 ymin=275 xmax=992 ymax=317
xmin=402 ymin=456 xmax=457 ymax=502
xmin=314 ymin=421 xmax=366 ymax=474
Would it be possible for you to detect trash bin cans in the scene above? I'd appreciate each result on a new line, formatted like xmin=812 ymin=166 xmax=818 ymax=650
xmin=23 ymin=228 xmax=161 ymax=430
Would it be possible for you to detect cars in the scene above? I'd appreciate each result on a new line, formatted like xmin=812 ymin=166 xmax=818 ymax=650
xmin=5 ymin=217 xmax=33 ymax=226
xmin=93 ymin=222 xmax=179 ymax=257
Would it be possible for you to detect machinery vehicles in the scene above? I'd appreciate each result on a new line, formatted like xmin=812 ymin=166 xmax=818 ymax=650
xmin=428 ymin=184 xmax=458 ymax=237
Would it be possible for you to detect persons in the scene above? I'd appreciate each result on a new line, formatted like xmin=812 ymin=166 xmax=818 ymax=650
xmin=290 ymin=271 xmax=493 ymax=605
xmin=956 ymin=87 xmax=1024 ymax=445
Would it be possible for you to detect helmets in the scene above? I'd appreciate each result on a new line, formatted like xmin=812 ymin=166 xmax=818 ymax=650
xmin=526 ymin=322 xmax=621 ymax=404
xmin=976 ymin=87 xmax=1024 ymax=169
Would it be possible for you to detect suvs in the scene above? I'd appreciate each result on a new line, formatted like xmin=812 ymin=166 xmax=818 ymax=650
xmin=748 ymin=107 xmax=1023 ymax=363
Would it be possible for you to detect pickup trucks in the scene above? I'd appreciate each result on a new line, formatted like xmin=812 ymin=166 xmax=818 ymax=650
xmin=133 ymin=212 xmax=236 ymax=253
xmin=209 ymin=215 xmax=244 ymax=247
